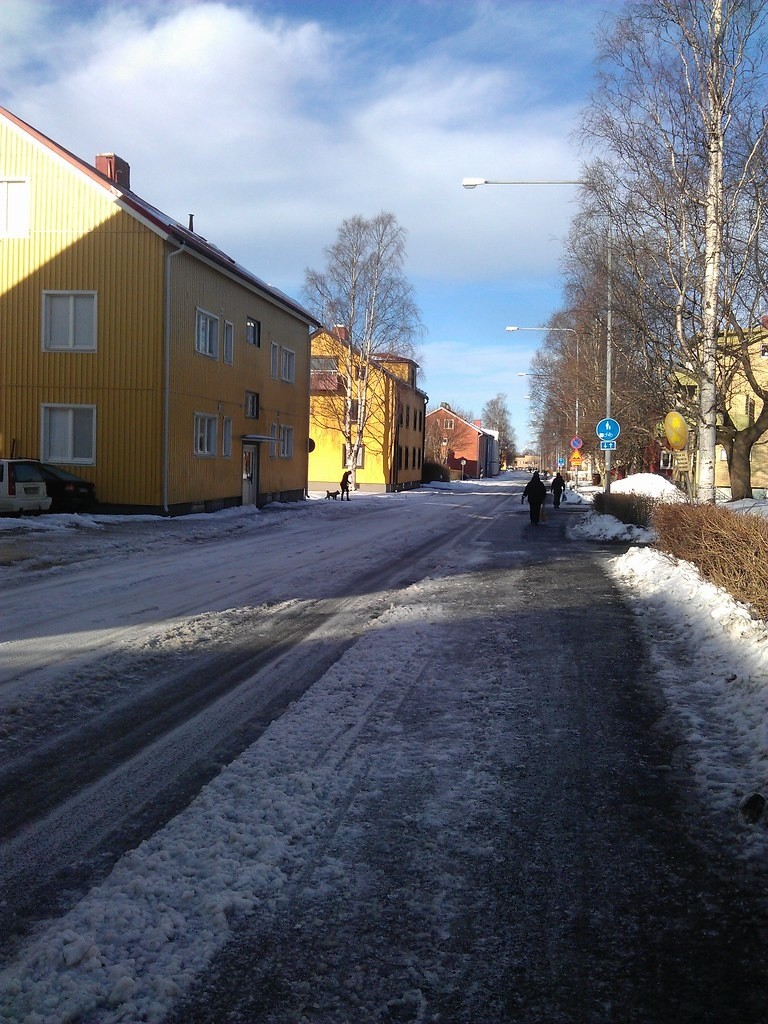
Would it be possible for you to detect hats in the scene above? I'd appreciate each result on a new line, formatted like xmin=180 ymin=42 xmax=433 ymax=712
xmin=533 ymin=471 xmax=539 ymax=475
xmin=348 ymin=471 xmax=352 ymax=474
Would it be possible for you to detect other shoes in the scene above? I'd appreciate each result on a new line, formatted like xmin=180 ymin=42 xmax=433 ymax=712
xmin=553 ymin=505 xmax=560 ymax=508
xmin=530 ymin=521 xmax=539 ymax=524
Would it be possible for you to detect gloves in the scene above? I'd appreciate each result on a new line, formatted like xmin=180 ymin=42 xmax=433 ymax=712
xmin=521 ymin=495 xmax=525 ymax=505
xmin=350 ymin=482 xmax=351 ymax=484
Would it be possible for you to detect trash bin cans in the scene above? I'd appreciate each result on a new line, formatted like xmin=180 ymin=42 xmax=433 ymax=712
xmin=592 ymin=473 xmax=600 ymax=485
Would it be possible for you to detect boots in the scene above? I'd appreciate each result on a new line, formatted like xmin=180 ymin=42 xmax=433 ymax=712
xmin=341 ymin=498 xmax=345 ymax=501
xmin=347 ymin=498 xmax=351 ymax=501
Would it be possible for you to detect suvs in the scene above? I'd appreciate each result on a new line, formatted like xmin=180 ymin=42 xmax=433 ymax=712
xmin=0 ymin=457 xmax=52 ymax=518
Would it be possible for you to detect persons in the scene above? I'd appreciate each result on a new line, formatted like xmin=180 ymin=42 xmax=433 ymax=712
xmin=522 ymin=471 xmax=546 ymax=525
xmin=340 ymin=471 xmax=352 ymax=501
xmin=551 ymin=473 xmax=565 ymax=508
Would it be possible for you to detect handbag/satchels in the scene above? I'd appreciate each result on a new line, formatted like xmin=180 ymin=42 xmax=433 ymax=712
xmin=562 ymin=493 xmax=567 ymax=502
xmin=541 ymin=510 xmax=546 ymax=522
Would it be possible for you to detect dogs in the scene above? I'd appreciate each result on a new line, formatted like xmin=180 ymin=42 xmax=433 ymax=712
xmin=324 ymin=490 xmax=340 ymax=501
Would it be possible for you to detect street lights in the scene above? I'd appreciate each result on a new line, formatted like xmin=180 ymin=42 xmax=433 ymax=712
xmin=519 ymin=373 xmax=575 ymax=480
xmin=464 ymin=178 xmax=613 ymax=514
xmin=505 ymin=327 xmax=579 ymax=492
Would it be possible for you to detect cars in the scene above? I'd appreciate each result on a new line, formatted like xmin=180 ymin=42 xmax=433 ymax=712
xmin=13 ymin=463 xmax=96 ymax=513
